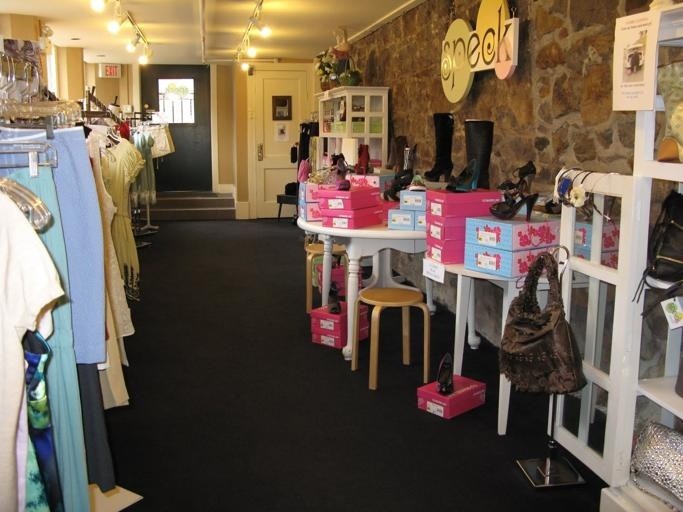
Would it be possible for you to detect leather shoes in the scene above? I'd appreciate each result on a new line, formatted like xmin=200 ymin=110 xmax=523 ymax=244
xmin=434 ymin=351 xmax=455 ymax=396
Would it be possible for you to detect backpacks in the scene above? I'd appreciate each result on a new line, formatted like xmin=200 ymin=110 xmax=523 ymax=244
xmin=645 ymin=187 xmax=683 ymax=283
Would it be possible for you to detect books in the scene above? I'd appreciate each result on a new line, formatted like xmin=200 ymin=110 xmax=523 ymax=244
xmin=611 ymin=10 xmax=662 ymax=114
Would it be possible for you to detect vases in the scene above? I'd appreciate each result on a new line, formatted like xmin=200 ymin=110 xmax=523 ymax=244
xmin=324 ymin=75 xmax=341 ymax=89
xmin=339 ymin=69 xmax=360 ymax=86
xmin=318 ymin=77 xmax=329 ymax=92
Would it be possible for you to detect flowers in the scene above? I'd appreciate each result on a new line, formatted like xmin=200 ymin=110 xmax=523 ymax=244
xmin=320 ymin=52 xmax=336 ymax=75
xmin=312 ymin=61 xmax=327 ymax=82
xmin=328 ymin=46 xmax=351 ymax=75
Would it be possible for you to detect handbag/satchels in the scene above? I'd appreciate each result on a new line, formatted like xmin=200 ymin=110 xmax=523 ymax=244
xmin=630 ymin=420 xmax=683 ymax=512
xmin=496 ymin=249 xmax=588 ymax=396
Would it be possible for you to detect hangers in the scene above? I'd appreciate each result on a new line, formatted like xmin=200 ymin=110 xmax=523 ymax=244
xmin=83 ymin=94 xmax=163 ymax=134
xmin=0 ymin=54 xmax=83 ymax=234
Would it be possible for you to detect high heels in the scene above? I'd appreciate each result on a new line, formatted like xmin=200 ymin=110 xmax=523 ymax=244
xmin=498 ymin=158 xmax=537 ymax=193
xmin=446 ymin=158 xmax=481 ymax=193
xmin=489 ymin=184 xmax=540 ymax=222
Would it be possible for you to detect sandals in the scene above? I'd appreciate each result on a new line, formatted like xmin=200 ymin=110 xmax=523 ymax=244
xmin=326 ymin=281 xmax=343 ymax=313
xmin=409 ymin=173 xmax=427 ymax=192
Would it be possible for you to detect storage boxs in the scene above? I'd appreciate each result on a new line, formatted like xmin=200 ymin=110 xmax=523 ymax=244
xmin=315 ymin=263 xmax=362 ymax=296
xmin=311 ymin=302 xmax=367 ymax=334
xmin=298 ymin=169 xmax=424 ymax=229
xmin=424 ymin=189 xmax=618 ymax=276
xmin=308 ymin=326 xmax=368 ymax=350
xmin=414 ymin=374 xmax=487 ymax=419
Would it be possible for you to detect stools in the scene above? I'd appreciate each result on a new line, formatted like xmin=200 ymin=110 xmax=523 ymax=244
xmin=353 ymin=286 xmax=432 ymax=391
xmin=304 ymin=243 xmax=350 ymax=313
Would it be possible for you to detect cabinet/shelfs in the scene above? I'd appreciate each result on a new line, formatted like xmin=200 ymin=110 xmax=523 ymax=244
xmin=316 ymin=84 xmax=389 ymax=166
xmin=546 ymin=1 xmax=683 ymax=511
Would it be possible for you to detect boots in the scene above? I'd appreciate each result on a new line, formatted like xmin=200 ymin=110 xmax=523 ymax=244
xmin=423 ymin=111 xmax=456 ymax=182
xmin=385 ymin=135 xmax=409 ymax=171
xmin=453 ymin=117 xmax=495 ymax=190
xmin=352 ymin=143 xmax=370 ymax=176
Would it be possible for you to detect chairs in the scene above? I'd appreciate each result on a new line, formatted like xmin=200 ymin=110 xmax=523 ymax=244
xmin=276 ymin=181 xmax=297 ymax=223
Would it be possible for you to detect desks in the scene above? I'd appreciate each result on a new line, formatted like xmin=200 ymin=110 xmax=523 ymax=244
xmin=294 ymin=214 xmax=437 ymax=361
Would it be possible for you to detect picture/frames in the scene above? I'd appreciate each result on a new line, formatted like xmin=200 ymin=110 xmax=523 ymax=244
xmin=272 ymin=96 xmax=292 ymax=121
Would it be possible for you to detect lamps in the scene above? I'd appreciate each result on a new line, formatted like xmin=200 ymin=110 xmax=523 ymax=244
xmin=231 ymin=0 xmax=270 ymax=73
xmin=92 ymin=0 xmax=152 ymax=69
